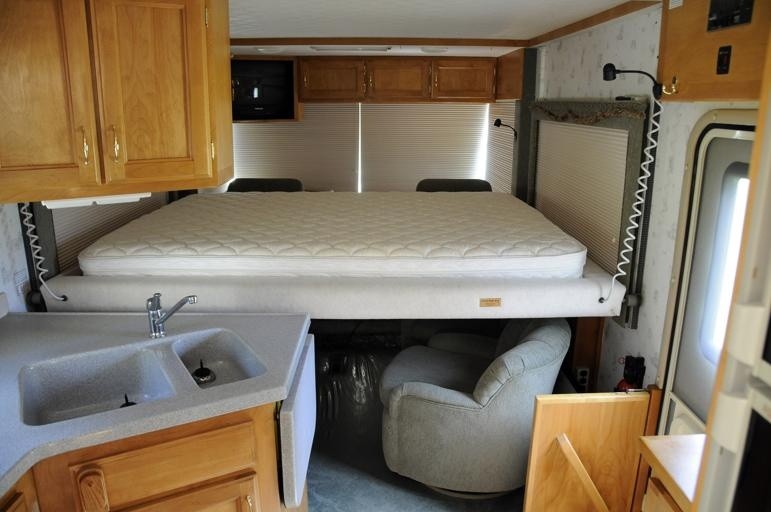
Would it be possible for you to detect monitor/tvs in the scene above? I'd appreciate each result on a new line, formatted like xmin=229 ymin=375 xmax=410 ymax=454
xmin=231 ymin=60 xmax=295 ymax=120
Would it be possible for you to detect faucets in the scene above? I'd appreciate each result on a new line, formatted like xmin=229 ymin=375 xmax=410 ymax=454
xmin=146 ymin=293 xmax=198 ymax=338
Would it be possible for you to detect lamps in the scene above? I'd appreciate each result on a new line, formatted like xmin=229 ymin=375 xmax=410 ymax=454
xmin=603 ymin=63 xmax=662 ymax=100
xmin=494 ymin=118 xmax=518 ymax=139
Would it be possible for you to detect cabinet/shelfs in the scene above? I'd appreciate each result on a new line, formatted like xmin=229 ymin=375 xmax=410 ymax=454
xmin=0 ymin=1 xmax=233 ymax=202
xmin=299 ymin=55 xmax=497 ymax=104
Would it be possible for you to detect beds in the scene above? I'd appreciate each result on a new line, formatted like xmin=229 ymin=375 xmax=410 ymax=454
xmin=41 ymin=191 xmax=626 ymax=319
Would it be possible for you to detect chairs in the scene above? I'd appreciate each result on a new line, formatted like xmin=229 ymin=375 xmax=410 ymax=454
xmin=378 ymin=318 xmax=571 ymax=499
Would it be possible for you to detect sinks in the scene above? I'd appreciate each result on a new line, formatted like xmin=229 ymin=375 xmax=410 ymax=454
xmin=19 ymin=344 xmax=176 ymax=425
xmin=171 ymin=327 xmax=270 ymax=390
